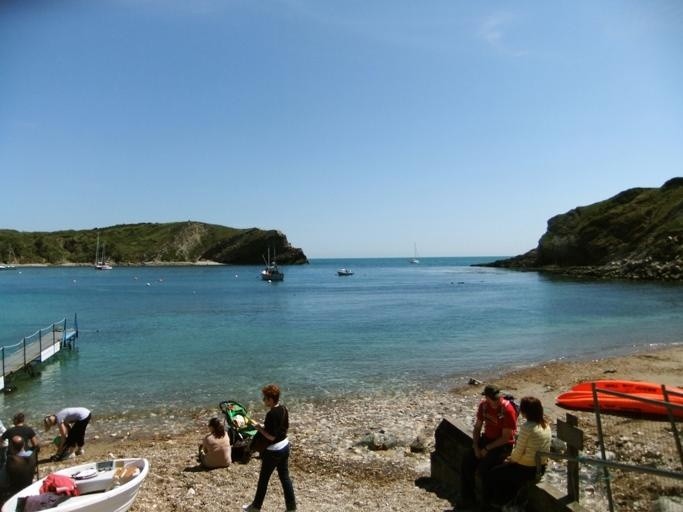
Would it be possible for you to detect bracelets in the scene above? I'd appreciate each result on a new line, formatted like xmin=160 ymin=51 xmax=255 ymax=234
xmin=484 ymin=446 xmax=489 ymax=452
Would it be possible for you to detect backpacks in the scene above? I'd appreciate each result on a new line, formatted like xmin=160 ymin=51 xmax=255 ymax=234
xmin=505 ymin=396 xmax=521 ymax=417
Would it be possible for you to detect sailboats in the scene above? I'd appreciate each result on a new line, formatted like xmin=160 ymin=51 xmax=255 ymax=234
xmin=94 ymin=232 xmax=114 ymax=269
xmin=262 ymin=247 xmax=284 ymax=280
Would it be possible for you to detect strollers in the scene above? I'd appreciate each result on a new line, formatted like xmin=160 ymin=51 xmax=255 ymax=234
xmin=219 ymin=399 xmax=261 ymax=460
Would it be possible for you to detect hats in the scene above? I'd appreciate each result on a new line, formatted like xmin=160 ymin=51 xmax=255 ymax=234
xmin=42 ymin=416 xmax=51 ymax=433
xmin=482 ymin=384 xmax=502 ymax=401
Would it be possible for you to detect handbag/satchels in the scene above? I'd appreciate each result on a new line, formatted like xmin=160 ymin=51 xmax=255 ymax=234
xmin=249 ymin=431 xmax=271 ymax=453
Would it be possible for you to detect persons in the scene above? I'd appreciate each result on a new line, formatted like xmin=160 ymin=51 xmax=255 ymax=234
xmin=447 ymin=384 xmax=519 ymax=512
xmin=474 ymin=395 xmax=554 ymax=511
xmin=42 ymin=406 xmax=93 ymax=460
xmin=234 ymin=415 xmax=247 ymax=427
xmin=240 ymin=384 xmax=298 ymax=511
xmin=0 ymin=412 xmax=40 ymax=503
xmin=197 ymin=417 xmax=233 ymax=467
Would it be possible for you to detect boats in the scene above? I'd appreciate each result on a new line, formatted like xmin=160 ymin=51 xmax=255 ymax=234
xmin=556 ymin=379 xmax=682 ymax=419
xmin=338 ymin=267 xmax=353 ymax=276
xmin=1 ymin=457 xmax=150 ymax=512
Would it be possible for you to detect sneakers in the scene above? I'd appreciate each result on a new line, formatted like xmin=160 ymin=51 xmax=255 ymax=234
xmin=64 ymin=447 xmax=85 ymax=459
xmin=242 ymin=505 xmax=260 ymax=512
